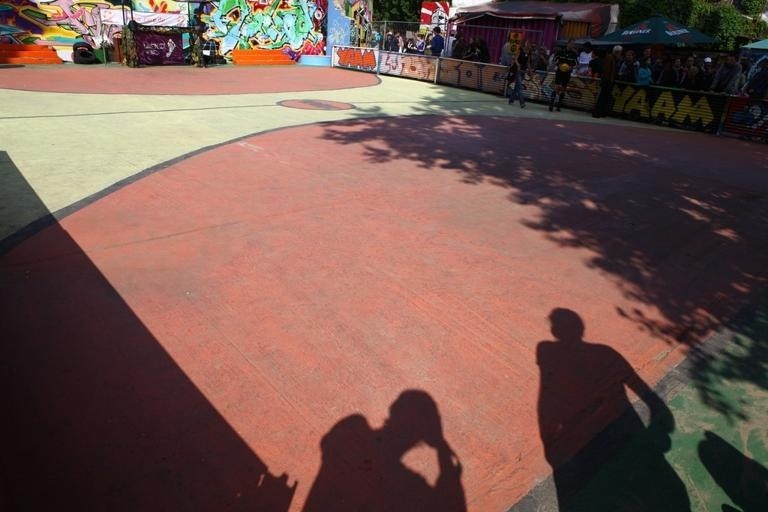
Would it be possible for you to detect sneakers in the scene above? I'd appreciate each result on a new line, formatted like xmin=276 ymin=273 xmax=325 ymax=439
xmin=549 ymin=106 xmax=563 ymax=113
xmin=508 ymin=99 xmax=528 ymax=109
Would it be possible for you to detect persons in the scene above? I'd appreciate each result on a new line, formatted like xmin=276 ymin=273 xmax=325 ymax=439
xmin=294 ymin=384 xmax=473 ymax=505
xmin=525 ymin=304 xmax=697 ymax=506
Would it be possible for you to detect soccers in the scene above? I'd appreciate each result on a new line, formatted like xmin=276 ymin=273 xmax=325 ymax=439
xmin=560 ymin=63 xmax=570 ymax=72
